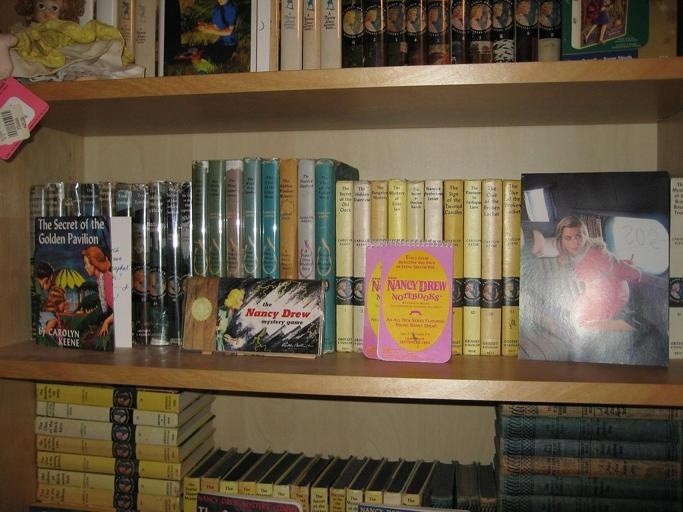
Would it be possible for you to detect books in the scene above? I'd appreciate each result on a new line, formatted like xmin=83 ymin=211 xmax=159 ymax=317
xmin=181 ymin=446 xmax=352 ymax=512
xmin=251 ymin=1 xmax=341 ymax=71
xmin=335 ymin=179 xmax=521 ymax=362
xmin=330 ymin=455 xmax=497 ymax=511
xmin=33 ymin=383 xmax=216 ymax=511
xmin=492 ymin=403 xmax=682 ymax=510
xmin=538 ymin=1 xmax=677 ymax=62
xmin=28 ymin=182 xmax=192 ymax=351
xmin=518 ymin=170 xmax=681 ymax=363
xmin=178 ymin=157 xmax=359 ymax=359
xmin=79 ymin=1 xmax=252 ymax=74
xmin=341 ymin=0 xmax=561 ymax=69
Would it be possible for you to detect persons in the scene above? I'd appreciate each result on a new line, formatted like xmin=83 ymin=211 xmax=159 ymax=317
xmin=556 ymin=216 xmax=655 ymax=333
xmin=0 ymin=1 xmax=145 ymax=78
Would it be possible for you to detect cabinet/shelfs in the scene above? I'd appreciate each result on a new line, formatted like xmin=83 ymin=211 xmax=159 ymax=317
xmin=0 ymin=0 xmax=683 ymax=512
